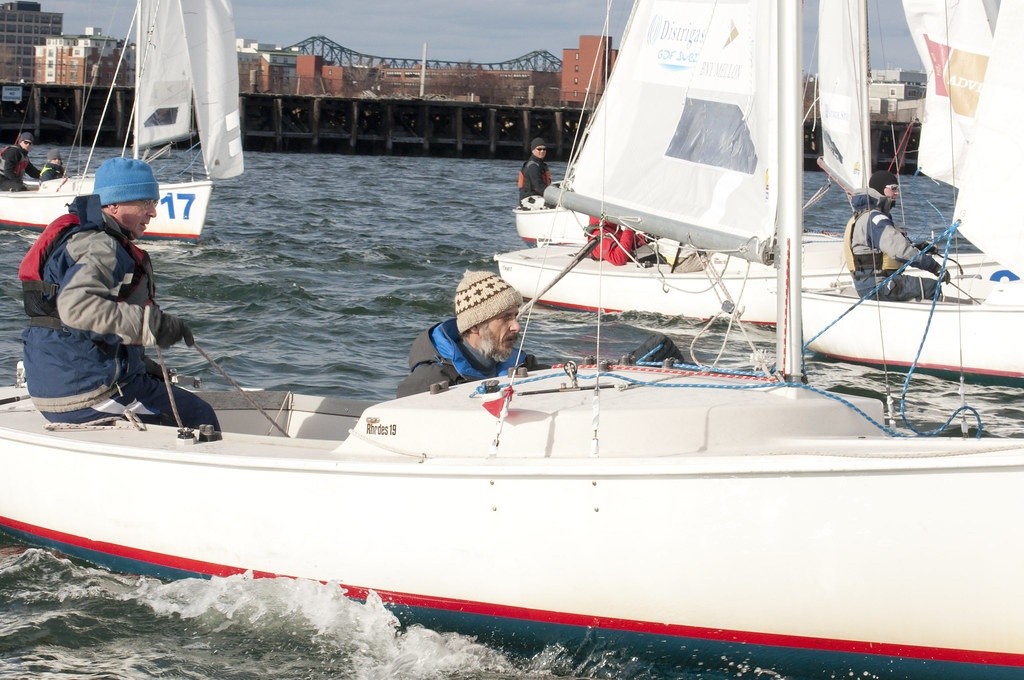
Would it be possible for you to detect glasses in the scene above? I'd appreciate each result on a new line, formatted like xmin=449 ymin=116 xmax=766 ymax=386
xmin=22 ymin=140 xmax=32 ymax=145
xmin=139 ymin=199 xmax=158 ymax=209
xmin=536 ymin=148 xmax=546 ymax=151
xmin=885 ymin=185 xmax=898 ymax=191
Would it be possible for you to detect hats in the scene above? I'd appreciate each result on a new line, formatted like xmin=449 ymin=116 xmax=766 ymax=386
xmin=531 ymin=137 xmax=546 ymax=150
xmin=455 ymin=270 xmax=523 ymax=333
xmin=47 ymin=149 xmax=60 ymax=161
xmin=93 ymin=157 xmax=160 ymax=206
xmin=18 ymin=132 xmax=34 ymax=143
xmin=869 ymin=171 xmax=898 ymax=196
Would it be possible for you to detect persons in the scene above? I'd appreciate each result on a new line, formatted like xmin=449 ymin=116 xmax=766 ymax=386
xmin=517 ymin=137 xmax=551 ymax=212
xmin=0 ymin=132 xmax=41 ymax=191
xmin=396 ymin=268 xmax=550 ymax=400
xmin=588 ymin=215 xmax=646 ymax=267
xmin=842 ymin=171 xmax=950 ymax=301
xmin=19 ymin=158 xmax=222 ymax=430
xmin=39 ymin=148 xmax=63 ymax=188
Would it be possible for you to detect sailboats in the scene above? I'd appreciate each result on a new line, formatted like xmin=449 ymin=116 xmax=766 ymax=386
xmin=493 ymin=0 xmax=1023 ymax=380
xmin=0 ymin=0 xmax=1024 ymax=679
xmin=0 ymin=0 xmax=248 ymax=239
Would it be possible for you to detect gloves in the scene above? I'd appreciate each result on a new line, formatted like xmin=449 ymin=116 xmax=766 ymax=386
xmin=933 ymin=264 xmax=950 ymax=285
xmin=631 ymin=334 xmax=684 ymax=367
xmin=157 ymin=313 xmax=194 ymax=349
xmin=915 ymin=241 xmax=938 ymax=254
xmin=151 ymin=362 xmax=173 ymax=381
